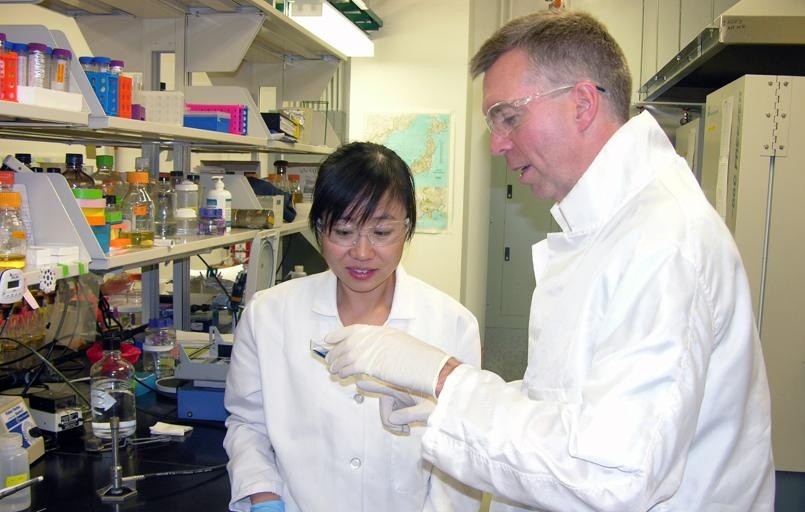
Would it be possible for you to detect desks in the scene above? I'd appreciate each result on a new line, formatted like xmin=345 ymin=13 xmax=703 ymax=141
xmin=0 ymin=338 xmax=236 ymax=512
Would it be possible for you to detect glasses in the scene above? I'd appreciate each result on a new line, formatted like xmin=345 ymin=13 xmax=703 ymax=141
xmin=483 ymin=83 xmax=605 ymax=137
xmin=317 ymin=219 xmax=410 ymax=248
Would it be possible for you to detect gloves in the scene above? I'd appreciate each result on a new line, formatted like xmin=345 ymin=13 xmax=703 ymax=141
xmin=324 ymin=324 xmax=454 ymax=402
xmin=356 ymin=381 xmax=436 ymax=435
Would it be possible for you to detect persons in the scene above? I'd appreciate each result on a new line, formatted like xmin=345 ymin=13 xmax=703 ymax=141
xmin=324 ymin=4 xmax=776 ymax=512
xmin=223 ymin=141 xmax=483 ymax=511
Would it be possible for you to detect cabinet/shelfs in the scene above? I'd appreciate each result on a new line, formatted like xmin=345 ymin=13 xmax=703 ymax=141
xmin=0 ymin=0 xmax=352 ymax=338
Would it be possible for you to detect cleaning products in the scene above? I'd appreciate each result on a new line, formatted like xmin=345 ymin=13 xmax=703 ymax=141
xmin=207 ymin=175 xmax=233 ymax=232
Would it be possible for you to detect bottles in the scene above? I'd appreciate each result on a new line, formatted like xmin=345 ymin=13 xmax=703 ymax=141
xmin=90 ymin=335 xmax=140 ymax=440
xmin=272 ymin=160 xmax=304 ymax=203
xmin=143 ymin=316 xmax=178 ymax=375
xmin=0 ymin=191 xmax=29 ymax=271
xmin=0 ymin=292 xmax=55 ymax=352
xmin=0 ymin=150 xmax=225 ymax=246
xmin=0 ymin=28 xmax=124 ymax=92
xmin=0 ymin=432 xmax=30 ymax=511
xmin=291 ymin=264 xmax=307 ymax=278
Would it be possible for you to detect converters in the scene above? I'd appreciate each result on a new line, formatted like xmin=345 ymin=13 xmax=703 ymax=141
xmin=29 ymin=389 xmax=76 ymax=414
